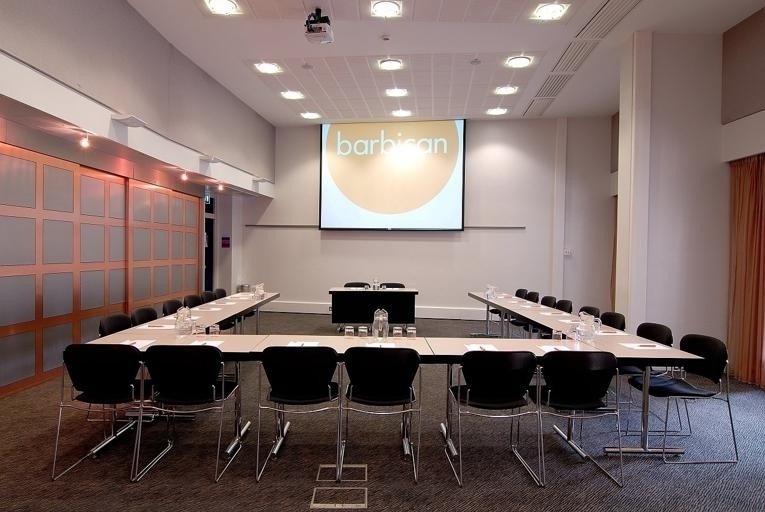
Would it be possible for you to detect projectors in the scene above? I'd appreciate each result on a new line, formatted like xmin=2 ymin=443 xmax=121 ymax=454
xmin=304 ymin=23 xmax=334 ymax=44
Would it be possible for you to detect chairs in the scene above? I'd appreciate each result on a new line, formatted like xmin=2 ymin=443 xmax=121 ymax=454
xmin=133 ymin=345 xmax=244 ymax=487
xmin=84 ymin=288 xmax=258 ymax=424
xmin=626 ymin=333 xmax=738 ymax=465
xmin=341 ymin=345 xmax=422 ymax=485
xmin=437 ymin=350 xmax=544 ymax=488
xmin=611 ymin=323 xmax=683 ymax=434
xmin=52 ymin=336 xmax=169 ymax=482
xmin=517 ymin=351 xmax=628 ymax=487
xmin=253 ymin=341 xmax=342 ymax=483
xmin=488 ymin=287 xmax=633 ymax=405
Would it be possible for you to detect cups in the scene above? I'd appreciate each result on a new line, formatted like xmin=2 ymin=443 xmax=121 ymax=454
xmin=344 ymin=326 xmax=355 ymax=340
xmin=393 ymin=326 xmax=404 ymax=342
xmin=485 ymin=284 xmax=496 ymax=300
xmin=365 ymin=285 xmax=369 ymax=290
xmin=249 ymin=293 xmax=261 ymax=301
xmin=552 ymin=329 xmax=562 ymax=344
xmin=407 ymin=327 xmax=416 ymax=343
xmin=194 ymin=320 xmax=205 ymax=337
xmin=566 ymin=327 xmax=578 ymax=347
xmin=382 ymin=285 xmax=386 ymax=289
xmin=210 ymin=324 xmax=219 ymax=338
xmin=357 ymin=325 xmax=368 ymax=341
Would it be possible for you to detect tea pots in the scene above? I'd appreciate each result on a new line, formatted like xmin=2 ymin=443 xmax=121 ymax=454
xmin=176 ymin=306 xmax=193 ymax=339
xmin=372 ymin=308 xmax=389 ymax=342
xmin=576 ymin=312 xmax=602 ymax=338
xmin=256 ymin=281 xmax=264 ymax=299
xmin=372 ymin=276 xmax=380 ymax=290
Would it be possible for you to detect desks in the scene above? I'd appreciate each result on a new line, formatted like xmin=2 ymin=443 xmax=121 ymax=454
xmin=467 ymin=289 xmax=703 ymax=456
xmin=84 ymin=288 xmax=281 ymax=419
xmin=327 ymin=285 xmax=420 ymax=333
